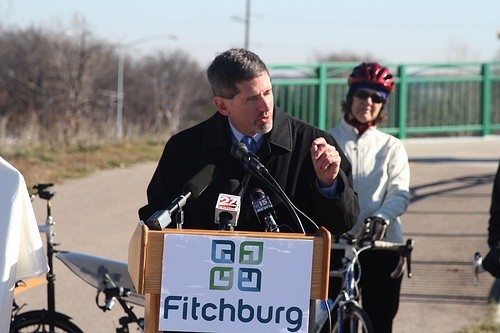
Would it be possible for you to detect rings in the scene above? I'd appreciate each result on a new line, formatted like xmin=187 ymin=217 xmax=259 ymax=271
xmin=329 ymin=151 xmax=332 ymax=156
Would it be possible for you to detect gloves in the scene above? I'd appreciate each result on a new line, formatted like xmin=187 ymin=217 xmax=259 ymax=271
xmin=369 ymin=216 xmax=388 ymax=241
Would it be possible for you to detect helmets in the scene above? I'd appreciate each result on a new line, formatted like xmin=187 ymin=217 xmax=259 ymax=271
xmin=348 ymin=62 xmax=394 ymax=94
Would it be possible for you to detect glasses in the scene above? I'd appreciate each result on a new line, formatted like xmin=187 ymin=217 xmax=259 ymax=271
xmin=354 ymin=90 xmax=385 ymax=104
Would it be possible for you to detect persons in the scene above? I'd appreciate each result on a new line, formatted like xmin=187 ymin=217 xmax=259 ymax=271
xmin=321 ymin=61 xmax=411 ymax=333
xmin=0 ymin=156 xmax=48 ymax=333
xmin=139 ymin=47 xmax=359 ymax=235
xmin=482 ymin=164 xmax=500 ymax=280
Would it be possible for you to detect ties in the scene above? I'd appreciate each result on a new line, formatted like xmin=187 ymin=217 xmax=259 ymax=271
xmin=241 ymin=137 xmax=255 ymax=173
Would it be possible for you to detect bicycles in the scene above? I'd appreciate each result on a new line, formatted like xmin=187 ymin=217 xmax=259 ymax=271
xmin=9 ymin=279 xmax=85 ymax=333
xmin=316 ymin=213 xmax=415 ymax=333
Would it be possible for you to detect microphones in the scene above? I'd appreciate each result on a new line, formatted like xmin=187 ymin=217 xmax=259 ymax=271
xmin=214 ymin=178 xmax=242 ymax=231
xmin=231 ymin=142 xmax=270 ymax=175
xmin=146 ymin=164 xmax=218 ymax=230
xmin=250 ymin=187 xmax=280 ymax=232
